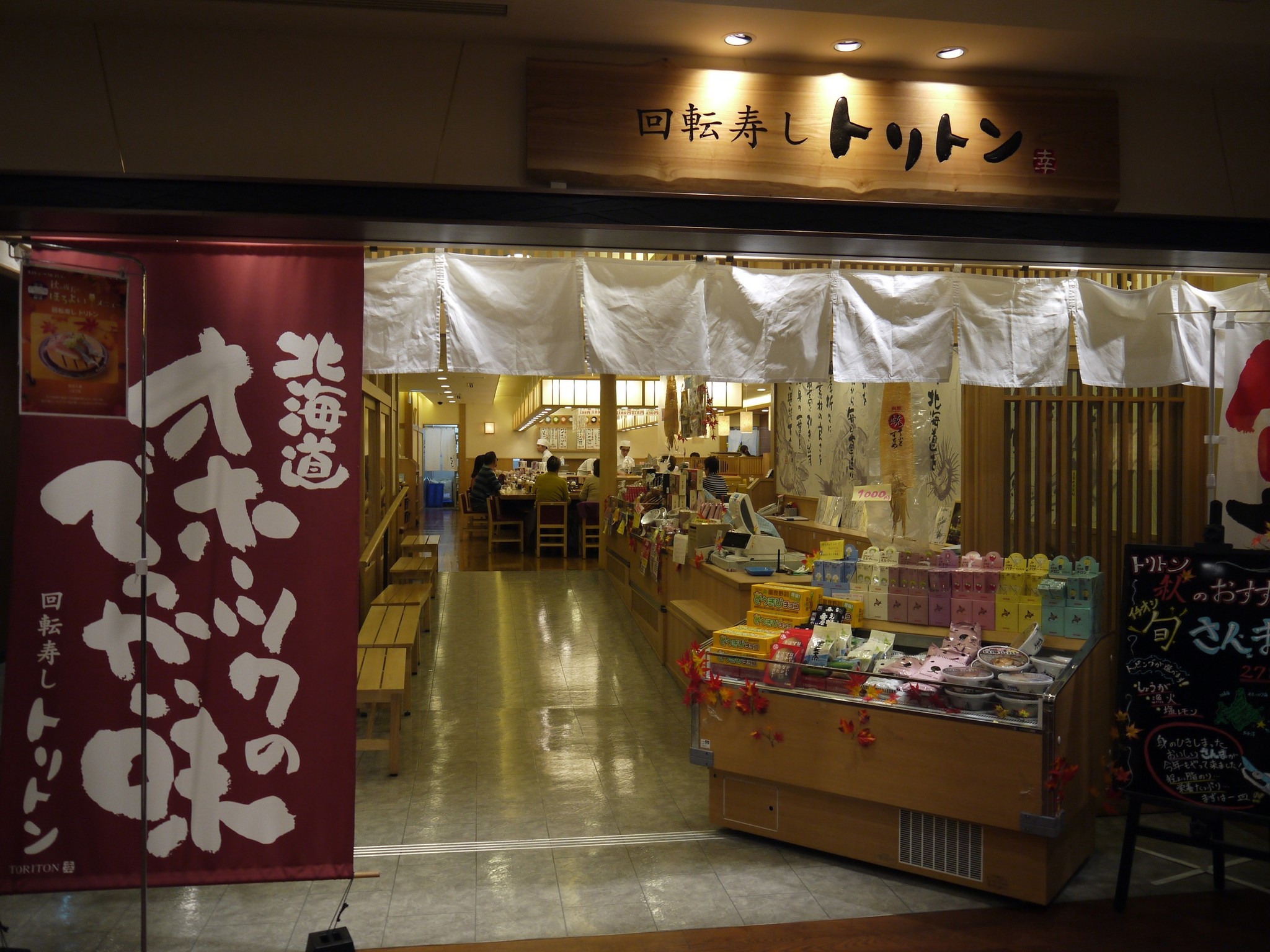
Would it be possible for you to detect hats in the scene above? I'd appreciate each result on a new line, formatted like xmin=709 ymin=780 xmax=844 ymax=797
xmin=620 ymin=440 xmax=631 ymax=447
xmin=537 ymin=438 xmax=549 ymax=447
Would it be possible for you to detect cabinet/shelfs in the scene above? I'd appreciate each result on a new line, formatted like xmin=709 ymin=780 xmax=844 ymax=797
xmin=773 ymin=524 xmax=873 ymax=558
xmin=381 ymin=457 xmax=420 ymax=532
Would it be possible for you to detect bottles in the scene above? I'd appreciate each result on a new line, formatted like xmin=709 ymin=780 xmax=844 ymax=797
xmin=511 ymin=469 xmax=544 ymax=494
xmin=561 ymin=456 xmax=564 ymax=466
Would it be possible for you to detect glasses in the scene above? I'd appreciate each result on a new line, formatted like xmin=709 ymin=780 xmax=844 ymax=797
xmin=621 ymin=448 xmax=630 ymax=452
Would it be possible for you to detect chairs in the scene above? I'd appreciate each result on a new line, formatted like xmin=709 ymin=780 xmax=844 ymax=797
xmin=487 ymin=494 xmax=528 ymax=553
xmin=577 ymin=501 xmax=599 ymax=559
xmin=457 ymin=487 xmax=495 ymax=542
xmin=534 ymin=502 xmax=567 ymax=559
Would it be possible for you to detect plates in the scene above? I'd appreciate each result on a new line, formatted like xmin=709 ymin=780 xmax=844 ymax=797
xmin=744 ymin=567 xmax=775 ymax=576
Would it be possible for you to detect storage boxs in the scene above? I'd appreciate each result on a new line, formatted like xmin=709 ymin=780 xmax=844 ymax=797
xmin=616 ymin=468 xmax=720 ymax=514
xmin=785 ymin=507 xmax=797 ymax=517
xmin=643 ymin=524 xmax=683 ymax=546
xmin=709 ymin=546 xmax=1114 ymax=697
xmin=688 ymin=523 xmax=729 ymax=560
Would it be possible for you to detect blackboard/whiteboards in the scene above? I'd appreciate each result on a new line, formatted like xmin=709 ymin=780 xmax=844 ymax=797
xmin=1110 ymin=542 xmax=1270 ymax=829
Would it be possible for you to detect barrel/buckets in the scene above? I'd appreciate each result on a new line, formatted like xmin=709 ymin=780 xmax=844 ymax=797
xmin=427 ymin=483 xmax=444 ymax=508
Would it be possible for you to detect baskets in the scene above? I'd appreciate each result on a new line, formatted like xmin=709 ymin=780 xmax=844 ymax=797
xmin=650 ymin=475 xmax=663 ymax=494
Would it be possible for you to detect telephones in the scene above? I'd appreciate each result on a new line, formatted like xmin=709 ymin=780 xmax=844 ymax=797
xmin=757 ymin=502 xmax=782 ymax=516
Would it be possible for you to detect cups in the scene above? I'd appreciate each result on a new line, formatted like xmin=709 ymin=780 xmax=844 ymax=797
xmin=785 ymin=508 xmax=797 ymax=516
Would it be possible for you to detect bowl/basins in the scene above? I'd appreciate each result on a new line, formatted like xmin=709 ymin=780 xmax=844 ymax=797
xmin=940 ymin=622 xmax=1072 ymax=718
xmin=640 ymin=507 xmax=689 ymax=528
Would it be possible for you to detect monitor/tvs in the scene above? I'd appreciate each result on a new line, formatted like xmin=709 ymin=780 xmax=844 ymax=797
xmin=729 ymin=492 xmax=760 ymax=535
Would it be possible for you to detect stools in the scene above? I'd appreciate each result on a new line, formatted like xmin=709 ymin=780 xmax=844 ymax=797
xmin=369 ymin=583 xmax=433 ymax=665
xmin=355 ymin=648 xmax=408 ymax=777
xmin=358 ymin=606 xmax=421 ymax=715
xmin=389 ymin=556 xmax=437 ymax=627
xmin=400 ymin=535 xmax=440 ymax=592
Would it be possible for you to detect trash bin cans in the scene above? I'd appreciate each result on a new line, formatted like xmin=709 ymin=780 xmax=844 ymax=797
xmin=426 ymin=482 xmax=444 ymax=508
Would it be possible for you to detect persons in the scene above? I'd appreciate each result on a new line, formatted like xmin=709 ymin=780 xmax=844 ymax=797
xmin=468 ymin=451 xmax=505 ymax=520
xmin=617 ymin=440 xmax=636 ymax=475
xmin=689 ymin=451 xmax=701 ymax=468
xmin=531 ymin=455 xmax=571 ymax=556
xmin=701 ymin=456 xmax=728 ymax=499
xmin=667 ymin=455 xmax=679 ymax=472
xmin=576 ymin=458 xmax=598 ymax=477
xmin=578 ymin=457 xmax=600 ymax=501
xmin=739 ymin=445 xmax=764 ymax=457
xmin=536 ymin=439 xmax=553 ymax=462
